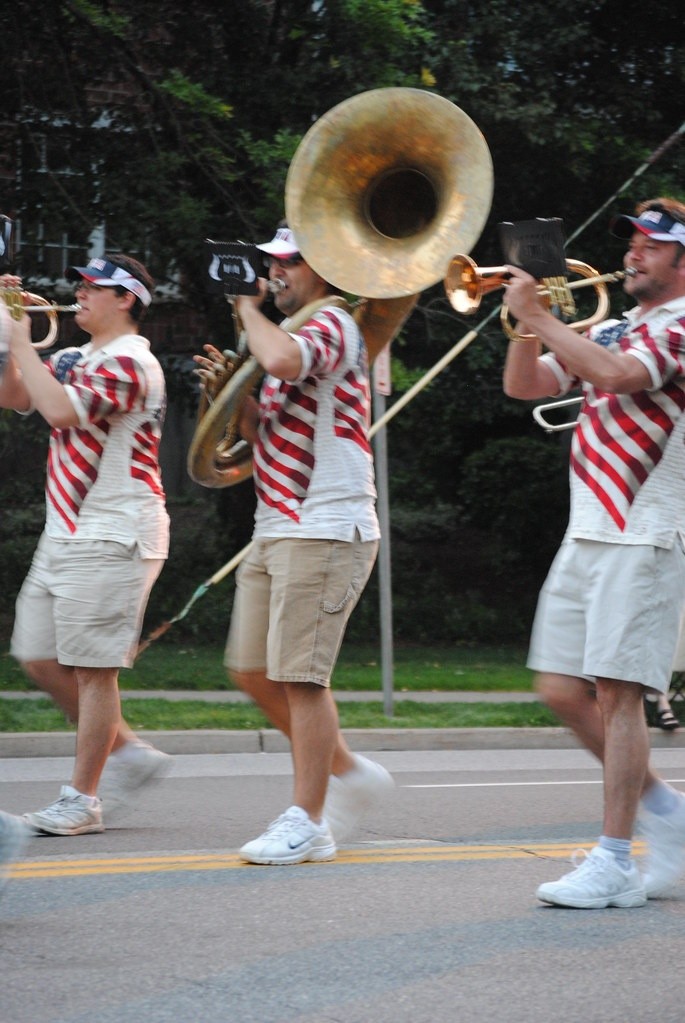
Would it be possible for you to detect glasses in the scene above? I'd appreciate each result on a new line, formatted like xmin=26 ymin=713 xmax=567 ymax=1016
xmin=73 ymin=282 xmax=126 ymax=295
xmin=261 ymin=254 xmax=312 ymax=270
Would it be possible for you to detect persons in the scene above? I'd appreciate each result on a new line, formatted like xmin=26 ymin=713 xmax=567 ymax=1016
xmin=0 ymin=254 xmax=170 ymax=838
xmin=192 ymin=220 xmax=391 ymax=865
xmin=652 ymin=692 xmax=679 ymax=728
xmin=501 ymin=197 xmax=684 ymax=909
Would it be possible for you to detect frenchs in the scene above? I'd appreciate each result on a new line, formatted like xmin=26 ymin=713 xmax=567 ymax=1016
xmin=186 ymin=86 xmax=495 ymax=489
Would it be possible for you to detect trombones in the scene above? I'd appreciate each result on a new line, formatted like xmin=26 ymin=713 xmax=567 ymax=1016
xmin=532 ymin=395 xmax=585 ymax=432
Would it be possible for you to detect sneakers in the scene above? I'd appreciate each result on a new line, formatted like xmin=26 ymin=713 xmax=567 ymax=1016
xmin=642 ymin=792 xmax=685 ymax=900
xmin=537 ymin=850 xmax=646 ymax=909
xmin=325 ymin=759 xmax=393 ymax=846
xmin=240 ymin=805 xmax=338 ymax=867
xmin=100 ymin=738 xmax=166 ymax=819
xmin=24 ymin=781 xmax=106 ymax=835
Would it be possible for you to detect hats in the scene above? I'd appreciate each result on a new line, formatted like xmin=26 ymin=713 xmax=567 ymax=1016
xmin=609 ymin=208 xmax=685 ymax=249
xmin=69 ymin=258 xmax=151 ymax=308
xmin=254 ymin=228 xmax=303 ymax=260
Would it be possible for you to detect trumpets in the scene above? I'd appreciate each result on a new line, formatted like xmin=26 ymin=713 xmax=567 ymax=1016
xmin=444 ymin=253 xmax=638 ymax=343
xmin=0 ymin=280 xmax=83 ymax=351
